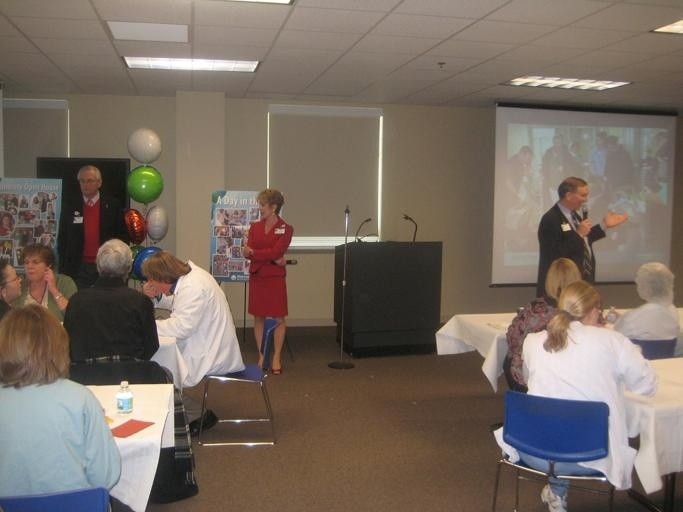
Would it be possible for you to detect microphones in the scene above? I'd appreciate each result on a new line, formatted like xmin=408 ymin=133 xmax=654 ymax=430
xmin=400 ymin=214 xmax=418 ymax=241
xmin=355 ymin=218 xmax=372 ymax=242
xmin=271 ymin=259 xmax=297 ymax=265
xmin=582 ymin=204 xmax=588 ymax=221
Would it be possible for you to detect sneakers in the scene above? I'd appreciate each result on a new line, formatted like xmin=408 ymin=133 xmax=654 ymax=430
xmin=540 ymin=482 xmax=567 ymax=511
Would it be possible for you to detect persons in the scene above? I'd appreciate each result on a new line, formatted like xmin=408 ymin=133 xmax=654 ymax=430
xmin=536 ymin=177 xmax=628 ymax=307
xmin=217 ymin=209 xmax=229 ymax=225
xmin=614 ymin=263 xmax=679 ymax=341
xmin=606 ymin=136 xmax=620 ymax=180
xmin=233 ymin=210 xmax=239 ymax=220
xmin=20 ymin=196 xmax=28 ymax=208
xmin=0 ymin=214 xmax=14 ymax=235
xmin=244 ymin=190 xmax=293 ymax=376
xmin=28 ymin=211 xmax=39 ymax=226
xmin=0 ymin=256 xmax=22 ymax=318
xmin=589 ymin=131 xmax=608 ymax=179
xmin=58 ymin=165 xmax=131 ymax=282
xmin=502 ymin=258 xmax=581 ymax=392
xmin=239 ymin=210 xmax=246 ymax=225
xmin=543 ymin=135 xmax=569 ymax=185
xmin=32 ymin=197 xmax=40 ymax=208
xmin=617 ymin=144 xmax=633 ymax=183
xmin=42 ymin=194 xmax=49 ymax=212
xmin=48 ymin=221 xmax=55 ymax=232
xmin=64 ymin=240 xmax=160 ymax=363
xmin=494 ymin=282 xmax=658 ymax=512
xmin=224 ymin=210 xmax=229 ymax=224
xmin=47 ymin=204 xmax=55 ymax=219
xmin=566 ymin=143 xmax=583 ymax=178
xmin=49 ymin=193 xmax=57 ymax=212
xmin=37 ymin=221 xmax=44 ymax=236
xmin=0 ymin=304 xmax=121 ymax=499
xmin=11 ymin=244 xmax=78 ymax=322
xmin=141 ymin=251 xmax=245 ymax=436
xmin=505 ymin=146 xmax=533 ymax=207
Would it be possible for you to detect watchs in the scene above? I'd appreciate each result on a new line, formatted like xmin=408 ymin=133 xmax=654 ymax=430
xmin=54 ymin=295 xmax=63 ymax=301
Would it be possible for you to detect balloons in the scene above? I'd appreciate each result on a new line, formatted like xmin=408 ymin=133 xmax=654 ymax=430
xmin=128 ymin=246 xmax=143 ymax=280
xmin=127 ymin=165 xmax=164 ymax=203
xmin=127 ymin=128 xmax=162 ymax=162
xmin=124 ymin=210 xmax=147 ymax=245
xmin=146 ymin=205 xmax=169 ymax=241
xmin=132 ymin=247 xmax=162 ymax=281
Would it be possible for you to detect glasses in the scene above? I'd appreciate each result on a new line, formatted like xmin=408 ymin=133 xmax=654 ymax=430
xmin=0 ymin=275 xmax=22 ymax=290
xmin=79 ymin=178 xmax=99 ymax=184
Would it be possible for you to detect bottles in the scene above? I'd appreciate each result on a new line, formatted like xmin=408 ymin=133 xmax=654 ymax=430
xmin=115 ymin=380 xmax=134 ymax=415
xmin=605 ymin=306 xmax=618 ymax=322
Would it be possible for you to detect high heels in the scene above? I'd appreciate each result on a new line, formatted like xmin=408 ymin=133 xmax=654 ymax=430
xmin=272 ymin=368 xmax=282 ymax=377
xmin=257 ymin=362 xmax=262 ymax=369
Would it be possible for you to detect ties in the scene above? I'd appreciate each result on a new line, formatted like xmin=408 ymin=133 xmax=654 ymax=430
xmin=572 ymin=212 xmax=593 ymax=279
xmin=87 ymin=200 xmax=93 ymax=206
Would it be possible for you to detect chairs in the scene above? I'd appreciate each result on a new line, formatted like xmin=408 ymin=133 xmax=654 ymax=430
xmin=492 ymin=391 xmax=610 ymax=512
xmin=630 ymin=337 xmax=678 ymax=360
xmin=199 ymin=318 xmax=283 ymax=447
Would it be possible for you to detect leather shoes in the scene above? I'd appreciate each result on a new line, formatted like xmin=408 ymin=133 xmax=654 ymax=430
xmin=190 ymin=412 xmax=219 ymax=434
